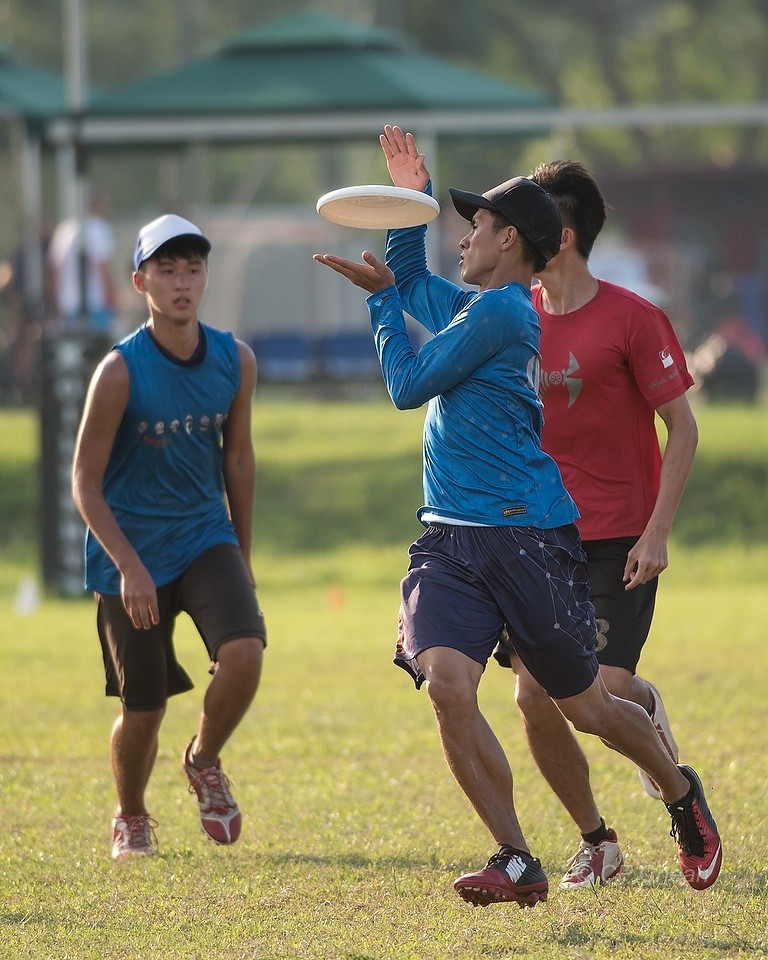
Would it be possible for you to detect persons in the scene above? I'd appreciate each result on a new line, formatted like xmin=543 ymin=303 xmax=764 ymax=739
xmin=54 ymin=181 xmax=118 ymax=348
xmin=310 ymin=125 xmax=723 ymax=904
xmin=71 ymin=211 xmax=268 ymax=862
xmin=499 ymin=161 xmax=700 ymax=894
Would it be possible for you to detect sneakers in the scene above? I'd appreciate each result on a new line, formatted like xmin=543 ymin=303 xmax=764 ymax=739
xmin=558 ymin=828 xmax=625 ymax=889
xmin=111 ymin=806 xmax=155 ymax=858
xmin=454 ymin=844 xmax=547 ymax=906
xmin=182 ymin=732 xmax=241 ymax=846
xmin=637 ymin=681 xmax=679 ymax=799
xmin=661 ymin=764 xmax=722 ymax=890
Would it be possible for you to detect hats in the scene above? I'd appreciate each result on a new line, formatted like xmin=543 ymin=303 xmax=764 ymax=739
xmin=449 ymin=176 xmax=562 ymax=273
xmin=133 ymin=214 xmax=211 ymax=272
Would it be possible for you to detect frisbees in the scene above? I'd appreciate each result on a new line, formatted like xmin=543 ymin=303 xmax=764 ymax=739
xmin=315 ymin=185 xmax=440 ymax=229
